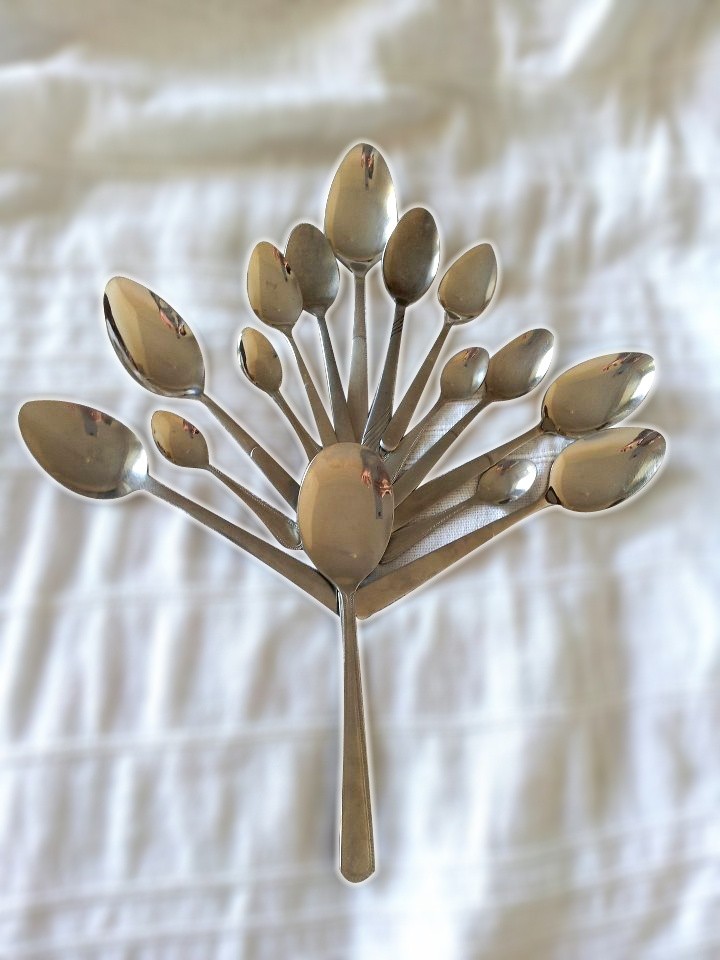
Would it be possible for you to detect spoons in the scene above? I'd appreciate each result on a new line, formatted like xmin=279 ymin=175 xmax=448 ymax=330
xmin=354 ymin=427 xmax=666 ymax=621
xmin=103 ymin=276 xmax=301 ymax=514
xmin=361 ymin=207 xmax=441 ymax=453
xmin=382 ymin=347 xmax=489 ymax=485
xmin=246 ymin=241 xmax=337 ymax=449
xmin=392 ymin=328 xmax=555 ymax=511
xmin=380 ymin=243 xmax=498 ymax=452
xmin=18 ymin=400 xmax=340 ymax=617
xmin=151 ymin=410 xmax=303 ymax=550
xmin=380 ymin=459 xmax=537 ymax=563
xmin=297 ymin=442 xmax=394 ymax=884
xmin=324 ymin=143 xmax=398 ymax=443
xmin=237 ymin=327 xmax=323 ymax=462
xmin=284 ymin=223 xmax=356 ymax=443
xmin=391 ymin=351 xmax=655 ymax=533
